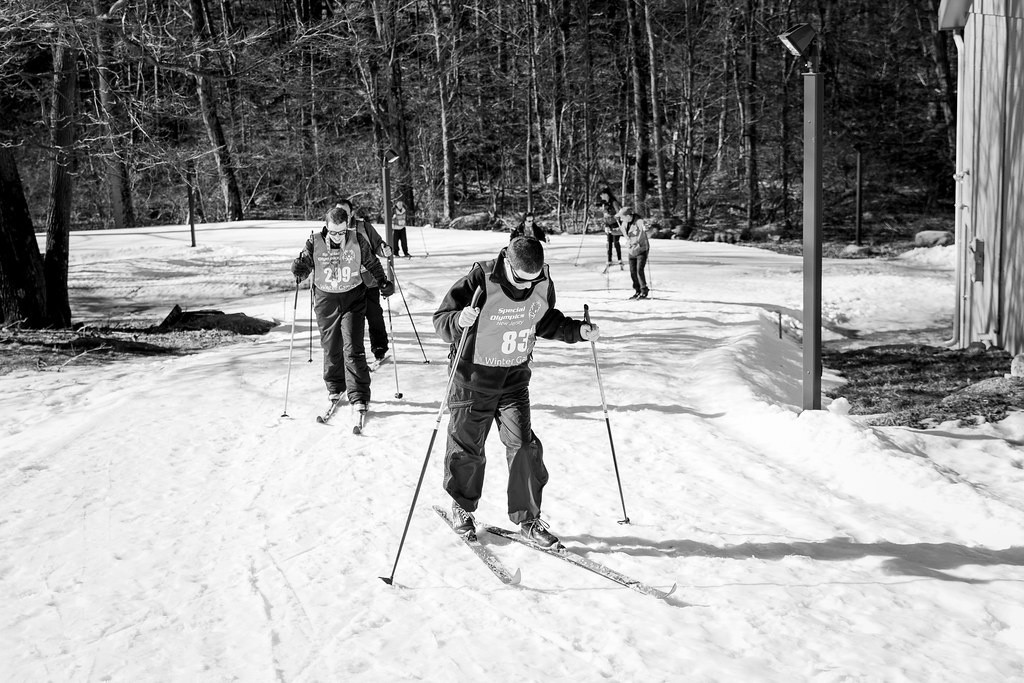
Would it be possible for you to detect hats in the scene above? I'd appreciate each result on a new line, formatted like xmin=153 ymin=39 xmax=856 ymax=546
xmin=614 ymin=207 xmax=635 ymax=218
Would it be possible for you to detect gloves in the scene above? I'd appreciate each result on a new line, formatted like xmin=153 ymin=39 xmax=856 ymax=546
xmin=291 ymin=257 xmax=308 ymax=277
xmin=377 ymin=276 xmax=394 ymax=297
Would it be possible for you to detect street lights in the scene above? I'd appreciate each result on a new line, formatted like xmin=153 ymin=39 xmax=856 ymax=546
xmin=383 ymin=149 xmax=399 ymax=247
xmin=779 ymin=24 xmax=821 ymax=408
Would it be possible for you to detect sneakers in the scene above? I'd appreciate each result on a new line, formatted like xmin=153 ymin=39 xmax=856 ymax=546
xmin=451 ymin=499 xmax=476 ymax=535
xmin=520 ymin=519 xmax=560 ymax=550
xmin=328 ymin=391 xmax=341 ymax=400
xmin=353 ymin=399 xmax=370 ymax=411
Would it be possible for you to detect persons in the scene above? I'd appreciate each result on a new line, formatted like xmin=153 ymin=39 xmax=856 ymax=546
xmin=591 ymin=188 xmax=623 ymax=264
xmin=510 ymin=213 xmax=551 ymax=244
xmin=615 ymin=207 xmax=650 ymax=301
xmin=336 ymin=199 xmax=393 ymax=359
xmin=433 ymin=236 xmax=600 ymax=550
xmin=391 ymin=201 xmax=412 ymax=258
xmin=291 ymin=206 xmax=394 ymax=411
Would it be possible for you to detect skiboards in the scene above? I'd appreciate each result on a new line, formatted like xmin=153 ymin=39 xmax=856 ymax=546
xmin=602 ymin=263 xmax=624 ymax=275
xmin=316 ymin=390 xmax=363 ymax=434
xmin=597 ymin=294 xmax=668 ymax=301
xmin=431 ymin=503 xmax=678 ymax=599
xmin=369 ymin=354 xmax=391 ymax=372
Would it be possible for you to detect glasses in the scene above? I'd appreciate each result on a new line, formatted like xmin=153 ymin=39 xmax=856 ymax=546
xmin=325 ymin=226 xmax=348 ymax=236
xmin=526 ymin=219 xmax=535 ymax=222
xmin=506 ymin=260 xmax=547 ymax=284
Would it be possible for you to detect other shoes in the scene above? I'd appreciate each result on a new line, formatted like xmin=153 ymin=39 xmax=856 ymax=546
xmin=607 ymin=260 xmax=612 ymax=266
xmin=373 ymin=346 xmax=385 ymax=361
xmin=618 ymin=258 xmax=622 ymax=264
xmin=629 ymin=289 xmax=641 ymax=300
xmin=637 ymin=286 xmax=650 ymax=301
